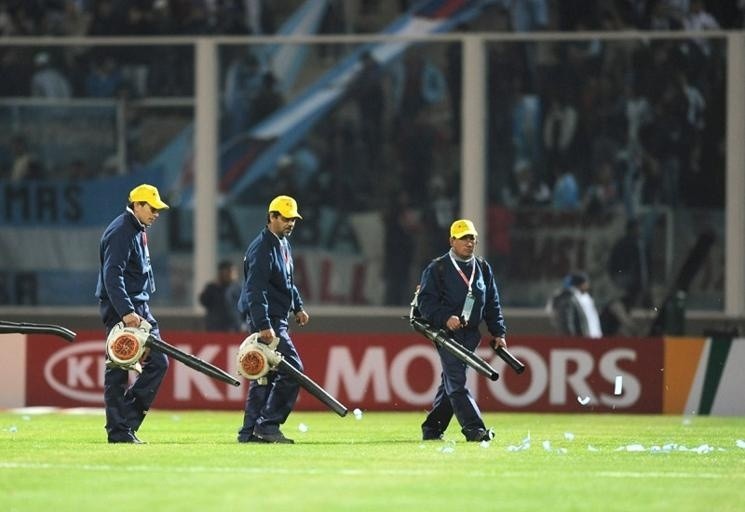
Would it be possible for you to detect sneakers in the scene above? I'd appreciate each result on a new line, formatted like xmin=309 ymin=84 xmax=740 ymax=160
xmin=423 ymin=424 xmax=442 ymax=439
xmin=238 ymin=424 xmax=293 ymax=443
xmin=105 ymin=421 xmax=147 ymax=443
xmin=466 ymin=429 xmax=495 ymax=441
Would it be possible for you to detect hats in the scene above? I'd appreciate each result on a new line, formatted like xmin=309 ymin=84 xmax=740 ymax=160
xmin=129 ymin=185 xmax=170 ymax=210
xmin=269 ymin=196 xmax=304 ymax=220
xmin=451 ymin=220 xmax=478 ymax=239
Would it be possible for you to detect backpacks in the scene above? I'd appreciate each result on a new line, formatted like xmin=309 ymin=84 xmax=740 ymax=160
xmin=410 ymin=257 xmax=490 ymax=332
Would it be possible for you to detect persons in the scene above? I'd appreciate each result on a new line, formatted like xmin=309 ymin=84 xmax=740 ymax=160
xmin=0 ymin=0 xmax=729 ymax=341
xmin=235 ymin=195 xmax=309 ymax=446
xmin=93 ymin=183 xmax=171 ymax=447
xmin=417 ymin=217 xmax=509 ymax=439
